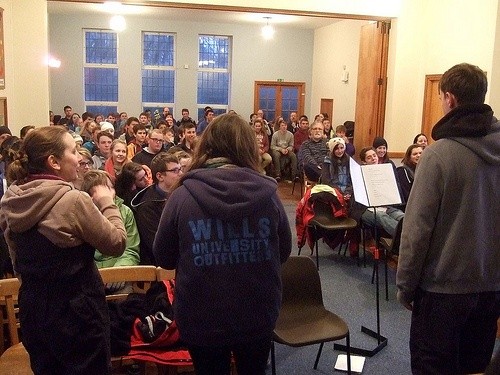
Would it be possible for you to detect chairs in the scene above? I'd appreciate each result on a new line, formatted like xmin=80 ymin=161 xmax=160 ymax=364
xmin=271 ymin=162 xmax=404 ymax=375
xmin=0 ymin=266 xmax=239 ymax=375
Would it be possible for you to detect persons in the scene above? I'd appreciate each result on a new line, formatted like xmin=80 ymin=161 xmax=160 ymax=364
xmin=250 ymin=107 xmax=427 ymax=238
xmin=152 ymin=114 xmax=292 ymax=374
xmin=397 ymin=63 xmax=500 ymax=375
xmin=1 ymin=125 xmax=35 ymax=200
xmin=49 ymin=103 xmax=216 ymax=324
xmin=1 ymin=127 xmax=127 ymax=375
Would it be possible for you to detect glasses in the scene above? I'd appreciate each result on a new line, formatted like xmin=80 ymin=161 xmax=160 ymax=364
xmin=150 ymin=137 xmax=164 ymax=144
xmin=160 ymin=167 xmax=183 ymax=174
xmin=79 ymin=160 xmax=94 ymax=168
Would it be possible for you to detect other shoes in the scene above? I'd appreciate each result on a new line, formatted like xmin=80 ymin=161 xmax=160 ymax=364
xmin=275 ymin=176 xmax=281 ymax=181
xmin=292 ymin=175 xmax=300 ymax=182
xmin=348 ymin=241 xmax=358 ymax=255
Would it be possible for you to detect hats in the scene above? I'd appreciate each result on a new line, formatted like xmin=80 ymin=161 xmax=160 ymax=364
xmin=326 ymin=137 xmax=346 ymax=151
xmin=373 ymin=136 xmax=387 ymax=150
xmin=100 ymin=121 xmax=115 ymax=132
xmin=69 ymin=130 xmax=83 ymax=143
xmin=0 ymin=124 xmax=12 ymax=138
